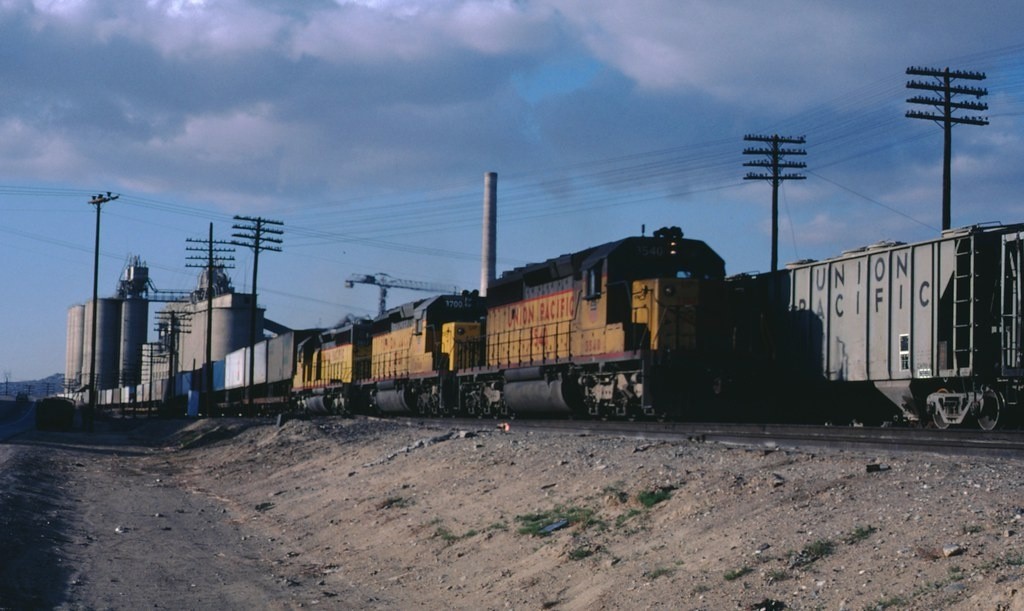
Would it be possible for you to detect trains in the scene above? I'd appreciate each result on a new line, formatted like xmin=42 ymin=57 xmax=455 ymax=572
xmin=665 ymin=218 xmax=1024 ymax=438
xmin=46 ymin=222 xmax=729 ymax=426
